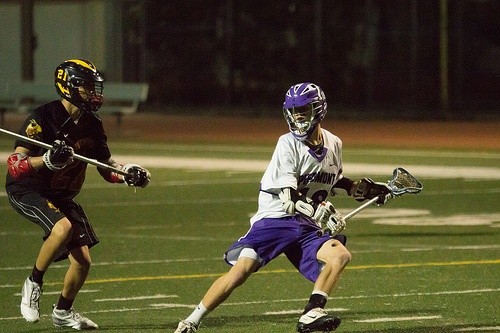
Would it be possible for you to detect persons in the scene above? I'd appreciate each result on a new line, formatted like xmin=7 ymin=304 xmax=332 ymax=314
xmin=170 ymin=81 xmax=399 ymax=332
xmin=6 ymin=54 xmax=151 ymax=330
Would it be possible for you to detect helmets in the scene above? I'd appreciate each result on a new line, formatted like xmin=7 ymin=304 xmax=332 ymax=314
xmin=283 ymin=83 xmax=327 ymax=141
xmin=55 ymin=59 xmax=104 ymax=113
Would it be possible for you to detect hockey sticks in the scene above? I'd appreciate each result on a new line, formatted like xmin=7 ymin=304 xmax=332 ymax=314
xmin=0 ymin=128 xmax=133 ymax=178
xmin=317 ymin=166 xmax=423 ymax=238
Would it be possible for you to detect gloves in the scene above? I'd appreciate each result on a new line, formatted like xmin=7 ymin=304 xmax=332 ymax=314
xmin=123 ymin=164 xmax=151 ymax=187
xmin=353 ymin=178 xmax=392 ymax=205
xmin=43 ymin=140 xmax=74 ymax=170
xmin=311 ymin=201 xmax=346 ymax=237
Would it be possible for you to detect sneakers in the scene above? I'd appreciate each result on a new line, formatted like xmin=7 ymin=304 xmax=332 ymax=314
xmin=174 ymin=320 xmax=202 ymax=333
xmin=297 ymin=307 xmax=341 ymax=333
xmin=52 ymin=304 xmax=99 ymax=330
xmin=20 ymin=276 xmax=43 ymax=322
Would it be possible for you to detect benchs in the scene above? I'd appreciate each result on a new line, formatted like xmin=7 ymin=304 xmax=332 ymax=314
xmin=0 ymin=78 xmax=150 ymax=137
